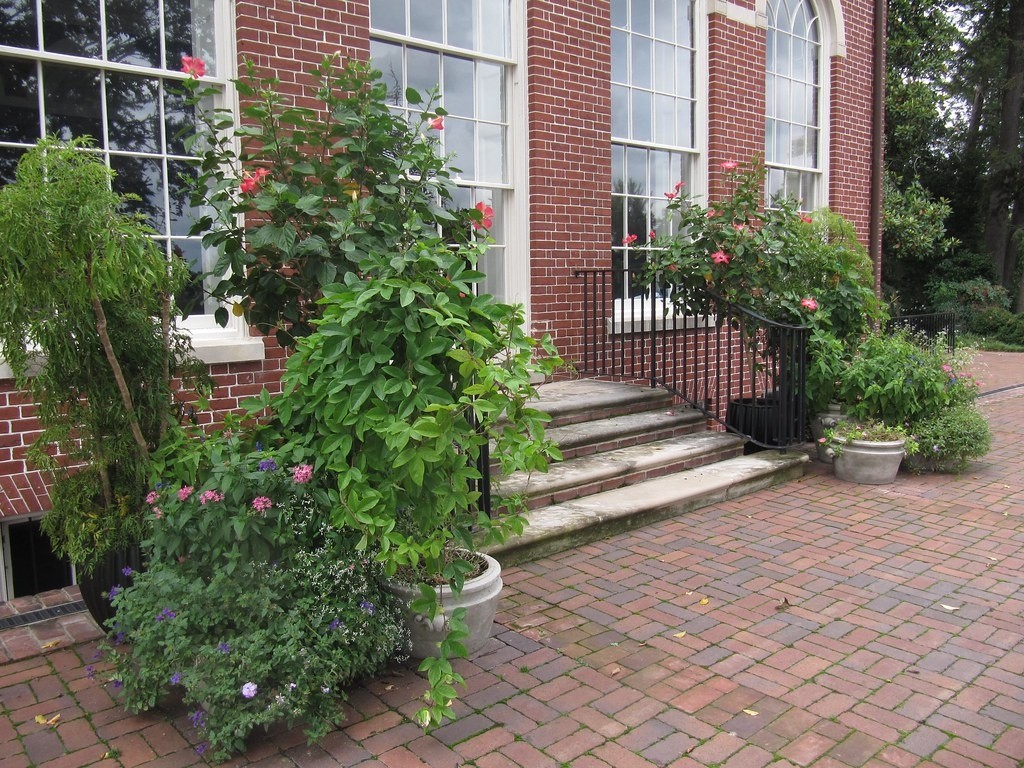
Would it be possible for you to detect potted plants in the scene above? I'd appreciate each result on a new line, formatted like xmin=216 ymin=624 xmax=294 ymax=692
xmin=1 ymin=129 xmax=216 ymax=642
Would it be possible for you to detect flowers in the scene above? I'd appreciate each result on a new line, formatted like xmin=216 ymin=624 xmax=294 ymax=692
xmin=147 ymin=252 xmax=577 ymax=734
xmin=82 ymin=429 xmax=413 ymax=764
xmin=905 ymin=407 xmax=993 ymax=484
xmin=623 ymin=151 xmax=863 ymax=404
xmin=816 ymin=418 xmax=919 ymax=458
xmin=793 ymin=329 xmax=952 ymax=426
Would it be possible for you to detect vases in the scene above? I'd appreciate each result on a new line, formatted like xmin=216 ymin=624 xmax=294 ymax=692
xmin=906 ymin=439 xmax=965 ymax=473
xmin=196 ymin=651 xmax=253 ymax=714
xmin=726 ymin=398 xmax=780 ymax=455
xmin=833 ymin=436 xmax=905 ymax=484
xmin=808 ymin=401 xmax=867 ymax=463
xmin=376 ymin=546 xmax=504 ymax=660
